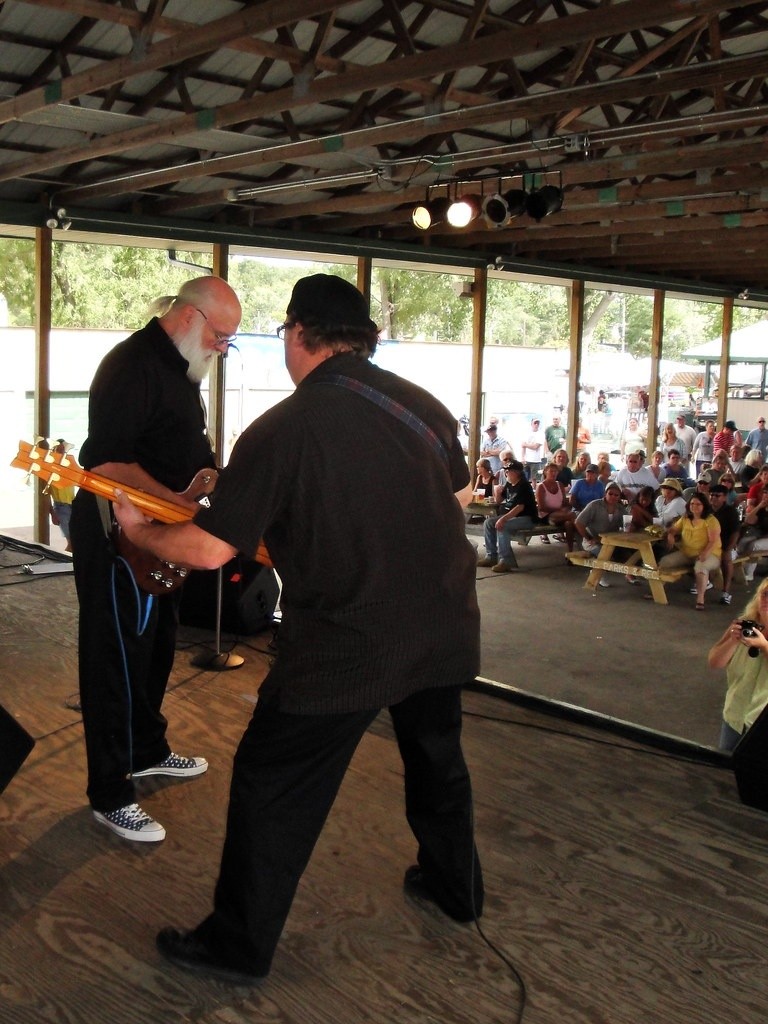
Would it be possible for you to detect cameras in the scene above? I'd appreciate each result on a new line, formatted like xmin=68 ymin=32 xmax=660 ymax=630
xmin=736 ymin=620 xmax=760 ymax=637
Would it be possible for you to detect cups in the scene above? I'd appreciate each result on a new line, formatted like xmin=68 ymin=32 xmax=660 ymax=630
xmin=477 ymin=489 xmax=486 ymax=500
xmin=653 ymin=517 xmax=663 ymax=526
xmin=623 ymin=515 xmax=633 ymax=532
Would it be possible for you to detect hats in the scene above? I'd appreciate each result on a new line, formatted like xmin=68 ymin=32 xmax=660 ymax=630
xmin=696 ymin=472 xmax=711 ymax=483
xmin=585 ymin=464 xmax=598 ymax=474
xmin=502 ymin=461 xmax=524 ymax=476
xmin=723 ymin=421 xmax=738 ymax=431
xmin=287 ymin=274 xmax=369 ymax=323
xmin=676 ymin=414 xmax=685 ymax=421
xmin=708 ymin=484 xmax=728 ymax=494
xmin=605 ymin=482 xmax=623 ymax=493
xmin=658 ymin=478 xmax=682 ymax=491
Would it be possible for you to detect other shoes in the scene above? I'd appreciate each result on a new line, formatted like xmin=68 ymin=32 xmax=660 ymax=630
xmin=477 ymin=556 xmax=496 ymax=566
xmin=491 ymin=562 xmax=509 ymax=573
xmin=157 ymin=925 xmax=265 ymax=982
xmin=402 ymin=863 xmax=483 ymax=925
xmin=536 ymin=531 xmax=756 ymax=615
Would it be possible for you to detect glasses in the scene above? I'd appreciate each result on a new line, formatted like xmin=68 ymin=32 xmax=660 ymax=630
xmin=608 ymin=491 xmax=618 ymax=498
xmin=721 ymin=479 xmax=734 ymax=483
xmin=193 ymin=305 xmax=240 ymax=348
xmin=660 ymin=485 xmax=673 ymax=490
xmin=699 ymin=481 xmax=708 ymax=485
xmin=709 ymin=494 xmax=722 ymax=498
xmin=628 ymin=460 xmax=638 ymax=463
xmin=758 ymin=421 xmax=766 ymax=424
xmin=275 ymin=323 xmax=294 ymax=336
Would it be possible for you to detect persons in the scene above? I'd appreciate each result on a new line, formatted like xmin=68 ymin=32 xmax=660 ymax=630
xmin=41 ymin=439 xmax=80 ymax=555
xmin=110 ymin=273 xmax=485 ymax=986
xmin=66 ymin=274 xmax=241 ymax=843
xmin=469 ymin=390 xmax=768 ymax=610
xmin=708 ymin=576 xmax=768 ymax=751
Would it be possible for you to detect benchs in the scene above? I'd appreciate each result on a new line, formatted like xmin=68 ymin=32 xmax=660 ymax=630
xmin=657 ymin=554 xmax=750 ymax=575
xmin=750 ymin=549 xmax=768 ymax=557
xmin=511 ymin=525 xmax=572 ymax=537
xmin=565 ymin=547 xmax=629 ymax=559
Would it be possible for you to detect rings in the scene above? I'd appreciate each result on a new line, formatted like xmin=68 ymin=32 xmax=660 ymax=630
xmin=731 ymin=629 xmax=734 ymax=633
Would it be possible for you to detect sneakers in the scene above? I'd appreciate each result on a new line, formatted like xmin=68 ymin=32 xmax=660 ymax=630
xmin=126 ymin=751 xmax=208 ymax=777
xmin=93 ymin=799 xmax=166 ymax=842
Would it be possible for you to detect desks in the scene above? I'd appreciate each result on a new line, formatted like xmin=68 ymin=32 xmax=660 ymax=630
xmin=463 ymin=502 xmax=576 ymax=553
xmin=569 ymin=527 xmax=749 ymax=605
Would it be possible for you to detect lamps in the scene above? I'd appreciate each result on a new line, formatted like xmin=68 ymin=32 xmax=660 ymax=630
xmin=494 ymin=264 xmax=505 ymax=273
xmin=51 ymin=205 xmax=67 ymax=219
xmin=446 ymin=181 xmax=486 ymax=228
xmin=412 ymin=184 xmax=452 ymax=230
xmin=523 ymin=171 xmax=565 ymax=224
xmin=742 ymin=294 xmax=750 ymax=300
xmin=489 ymin=254 xmax=502 ymax=264
xmin=741 ymin=287 xmax=749 ymax=294
xmin=736 ymin=289 xmax=745 ymax=298
xmin=480 ymin=175 xmax=529 ymax=230
xmin=486 ymin=258 xmax=495 ymax=271
xmin=55 ymin=218 xmax=72 ymax=231
xmin=44 ymin=213 xmax=58 ymax=229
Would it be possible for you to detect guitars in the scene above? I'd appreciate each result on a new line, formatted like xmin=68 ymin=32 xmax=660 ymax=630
xmin=11 ymin=438 xmax=276 ymax=565
xmin=114 ymin=467 xmax=221 ymax=597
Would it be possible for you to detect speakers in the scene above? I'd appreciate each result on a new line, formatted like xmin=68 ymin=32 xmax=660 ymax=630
xmin=206 ymin=550 xmax=280 ymax=636
